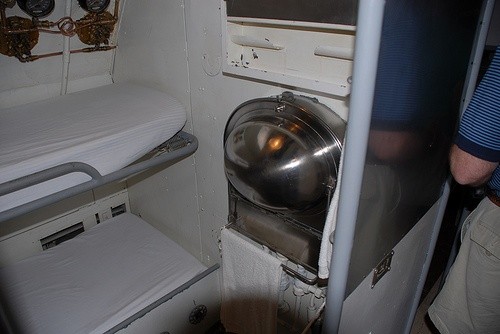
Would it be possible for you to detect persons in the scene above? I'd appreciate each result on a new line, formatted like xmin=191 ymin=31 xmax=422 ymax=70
xmin=340 ymin=0 xmax=440 ymax=304
xmin=426 ymin=38 xmax=500 ymax=334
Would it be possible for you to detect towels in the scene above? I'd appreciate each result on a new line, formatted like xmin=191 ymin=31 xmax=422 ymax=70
xmin=219 ymin=228 xmax=284 ymax=334
xmin=318 ymin=124 xmax=347 ymax=279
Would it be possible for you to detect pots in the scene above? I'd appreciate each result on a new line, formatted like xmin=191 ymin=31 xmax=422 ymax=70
xmin=222 ymin=99 xmax=343 ymax=217
